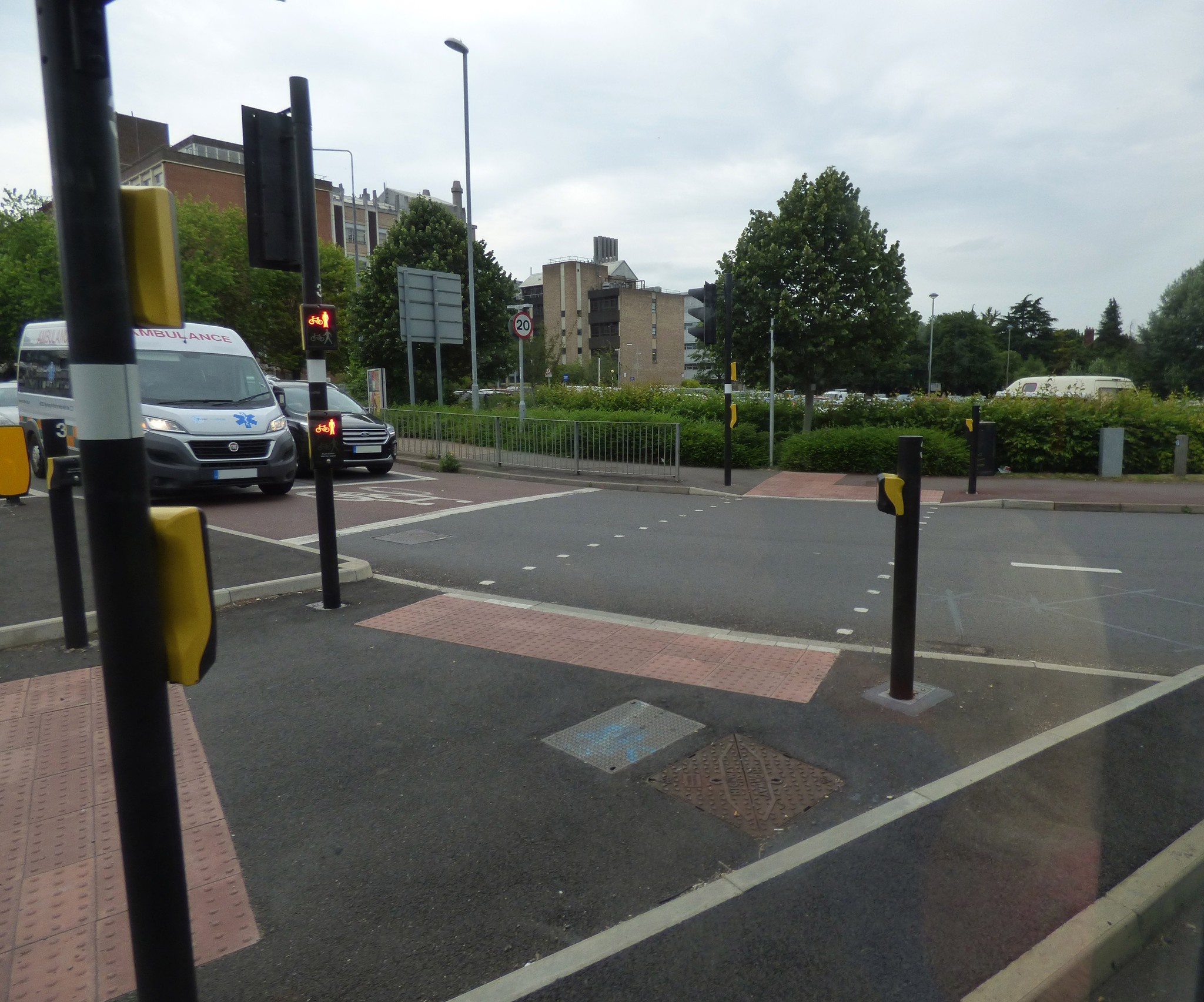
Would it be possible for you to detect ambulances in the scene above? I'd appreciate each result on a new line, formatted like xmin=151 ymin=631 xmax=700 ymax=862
xmin=16 ymin=319 xmax=298 ymax=499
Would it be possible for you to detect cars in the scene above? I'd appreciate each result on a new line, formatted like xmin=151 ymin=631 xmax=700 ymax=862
xmin=661 ymin=385 xmax=980 ymax=413
xmin=452 ymin=385 xmax=530 ymax=404
xmin=0 ymin=381 xmax=20 ymax=426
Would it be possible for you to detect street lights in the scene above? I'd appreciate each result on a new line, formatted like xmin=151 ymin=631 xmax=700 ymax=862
xmin=506 ymin=303 xmax=533 ymax=434
xmin=924 ymin=293 xmax=939 ymax=399
xmin=613 ymin=349 xmax=620 ymax=388
xmin=1005 ymin=325 xmax=1014 ymax=387
xmin=445 ymin=36 xmax=481 ymax=414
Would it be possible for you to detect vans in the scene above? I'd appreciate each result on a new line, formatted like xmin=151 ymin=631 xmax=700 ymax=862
xmin=994 ymin=375 xmax=1140 ymax=404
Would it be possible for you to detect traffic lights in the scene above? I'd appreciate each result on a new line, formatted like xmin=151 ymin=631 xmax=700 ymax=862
xmin=299 ymin=304 xmax=339 ymax=350
xmin=688 ymin=281 xmax=717 ymax=347
xmin=306 ymin=410 xmax=343 ymax=468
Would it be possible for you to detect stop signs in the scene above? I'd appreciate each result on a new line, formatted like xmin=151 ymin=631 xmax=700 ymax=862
xmin=514 ymin=312 xmax=533 ymax=338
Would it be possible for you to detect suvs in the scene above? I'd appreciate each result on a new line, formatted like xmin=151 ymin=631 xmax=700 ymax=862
xmin=245 ymin=376 xmax=397 ymax=477
